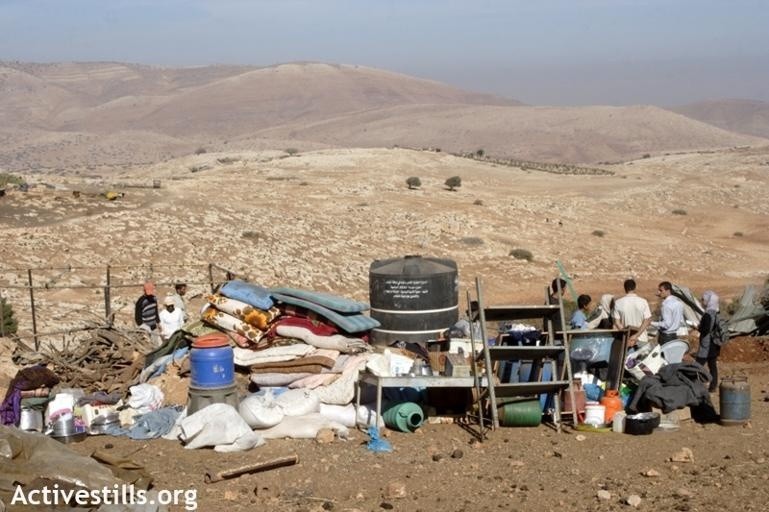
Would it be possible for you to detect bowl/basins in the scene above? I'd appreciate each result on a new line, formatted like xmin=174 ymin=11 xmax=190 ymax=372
xmin=46 ymin=426 xmax=90 ymax=443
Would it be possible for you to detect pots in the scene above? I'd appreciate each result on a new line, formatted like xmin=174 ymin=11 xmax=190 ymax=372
xmin=91 ymin=412 xmax=122 ymax=434
xmin=49 ymin=415 xmax=78 ymax=435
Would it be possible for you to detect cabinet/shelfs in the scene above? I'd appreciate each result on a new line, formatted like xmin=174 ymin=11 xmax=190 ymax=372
xmin=465 ymin=275 xmax=578 ymax=442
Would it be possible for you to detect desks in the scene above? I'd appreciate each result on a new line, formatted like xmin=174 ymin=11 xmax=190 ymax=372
xmin=352 ymin=368 xmax=500 ymax=432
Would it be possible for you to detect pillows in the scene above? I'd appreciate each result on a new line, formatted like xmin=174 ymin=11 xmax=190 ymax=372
xmin=201 ymin=281 xmax=280 ymax=345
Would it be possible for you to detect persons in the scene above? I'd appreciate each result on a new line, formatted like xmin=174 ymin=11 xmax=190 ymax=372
xmin=610 ymin=278 xmax=651 ymax=354
xmin=543 ymin=278 xmax=568 ymax=330
xmin=170 ymin=281 xmax=190 ymax=323
xmin=135 ymin=282 xmax=162 ymax=334
xmin=569 ymin=293 xmax=593 ymax=333
xmin=650 ymin=281 xmax=685 ymax=359
xmin=591 ymin=293 xmax=615 ymax=329
xmin=689 ymin=289 xmax=723 ymax=397
xmin=157 ymin=296 xmax=184 ymax=340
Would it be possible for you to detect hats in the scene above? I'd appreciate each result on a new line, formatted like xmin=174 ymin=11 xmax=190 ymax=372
xmin=144 ymin=282 xmax=153 ymax=296
xmin=163 ymin=298 xmax=174 ymax=306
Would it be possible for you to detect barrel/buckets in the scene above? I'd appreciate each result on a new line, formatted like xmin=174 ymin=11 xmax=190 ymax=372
xmin=189 ymin=334 xmax=234 ymax=388
xmin=487 ymin=395 xmax=543 ymax=427
xmin=187 ymin=390 xmax=239 ymax=418
xmin=382 ymin=400 xmax=424 ymax=433
xmin=585 ymin=404 xmax=605 ymax=425
xmin=719 ymin=374 xmax=751 ymax=421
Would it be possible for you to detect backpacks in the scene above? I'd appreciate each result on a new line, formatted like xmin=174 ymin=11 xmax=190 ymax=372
xmin=706 ymin=311 xmax=729 ymax=346
